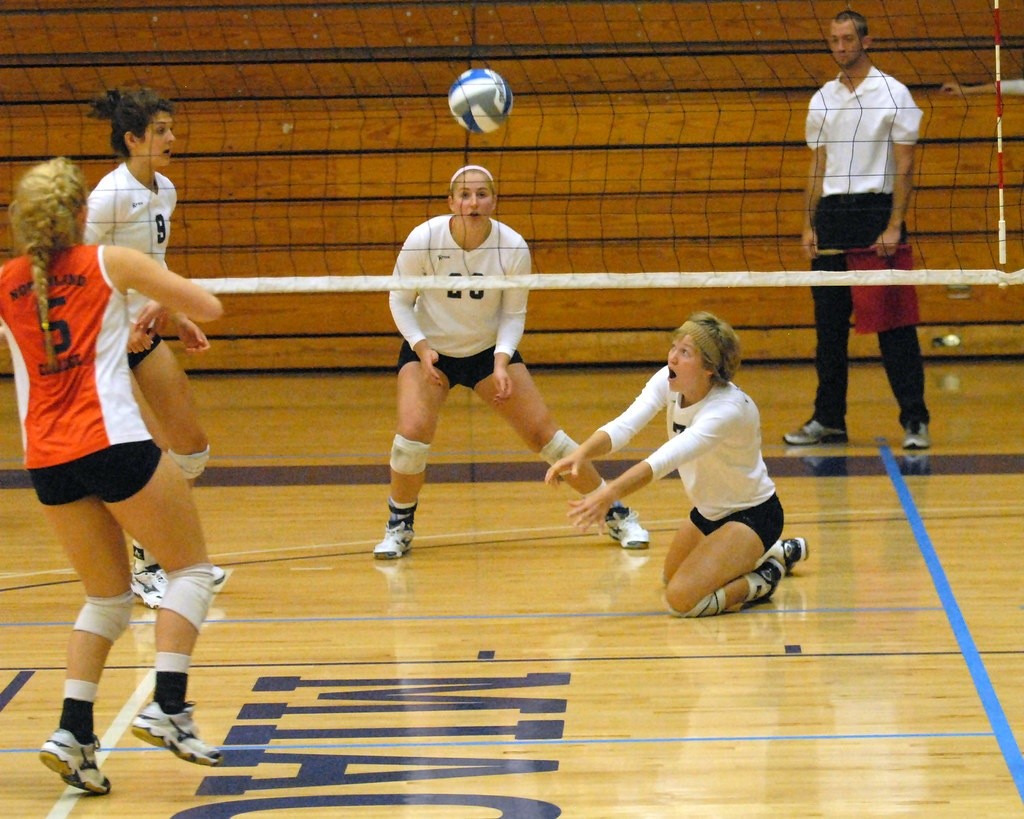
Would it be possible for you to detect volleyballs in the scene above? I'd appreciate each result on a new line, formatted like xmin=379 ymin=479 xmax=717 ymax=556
xmin=446 ymin=67 xmax=515 ymax=134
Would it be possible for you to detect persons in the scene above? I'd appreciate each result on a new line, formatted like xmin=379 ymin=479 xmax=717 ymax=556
xmin=79 ymin=84 xmax=229 ymax=613
xmin=942 ymin=79 xmax=1024 ymax=96
xmin=372 ymin=163 xmax=649 ymax=560
xmin=544 ymin=309 xmax=808 ymax=617
xmin=782 ymin=9 xmax=932 ymax=449
xmin=0 ymin=154 xmax=225 ymax=794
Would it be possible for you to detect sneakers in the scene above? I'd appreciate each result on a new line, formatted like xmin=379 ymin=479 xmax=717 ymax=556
xmin=212 ymin=566 xmax=226 ymax=586
xmin=604 ymin=507 xmax=649 ymax=548
xmin=131 ymin=568 xmax=169 ymax=607
xmin=781 ymin=536 xmax=809 ymax=574
xmin=901 ymin=419 xmax=929 ymax=448
xmin=751 ymin=555 xmax=785 ymax=600
xmin=132 ymin=700 xmax=223 ymax=767
xmin=373 ymin=520 xmax=414 ymax=559
xmin=38 ymin=728 xmax=111 ymax=794
xmin=781 ymin=417 xmax=847 ymax=446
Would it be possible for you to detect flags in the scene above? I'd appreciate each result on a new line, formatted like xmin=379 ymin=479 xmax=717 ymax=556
xmin=846 ymin=250 xmax=922 ymax=334
